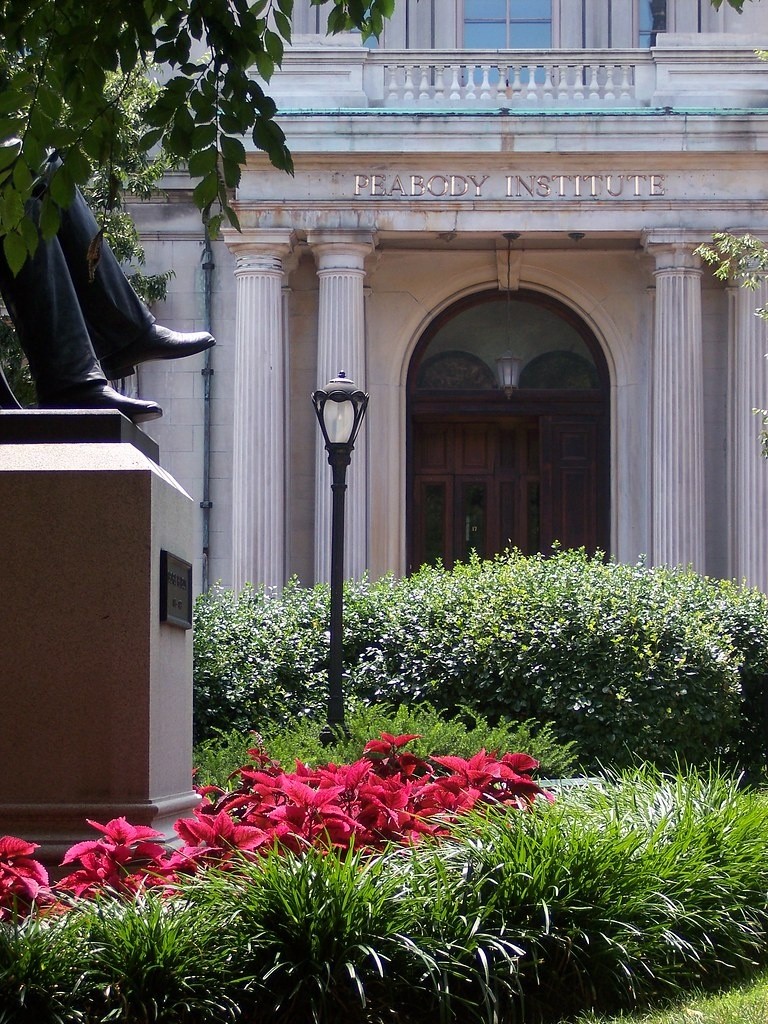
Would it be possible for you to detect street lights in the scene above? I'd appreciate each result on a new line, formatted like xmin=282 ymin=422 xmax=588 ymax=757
xmin=309 ymin=369 xmax=372 ymax=746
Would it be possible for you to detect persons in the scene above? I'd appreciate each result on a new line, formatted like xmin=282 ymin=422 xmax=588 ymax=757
xmin=0 ymin=140 xmax=217 ymax=421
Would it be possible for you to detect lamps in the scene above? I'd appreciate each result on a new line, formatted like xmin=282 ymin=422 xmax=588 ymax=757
xmin=493 ymin=288 xmax=522 ymax=401
